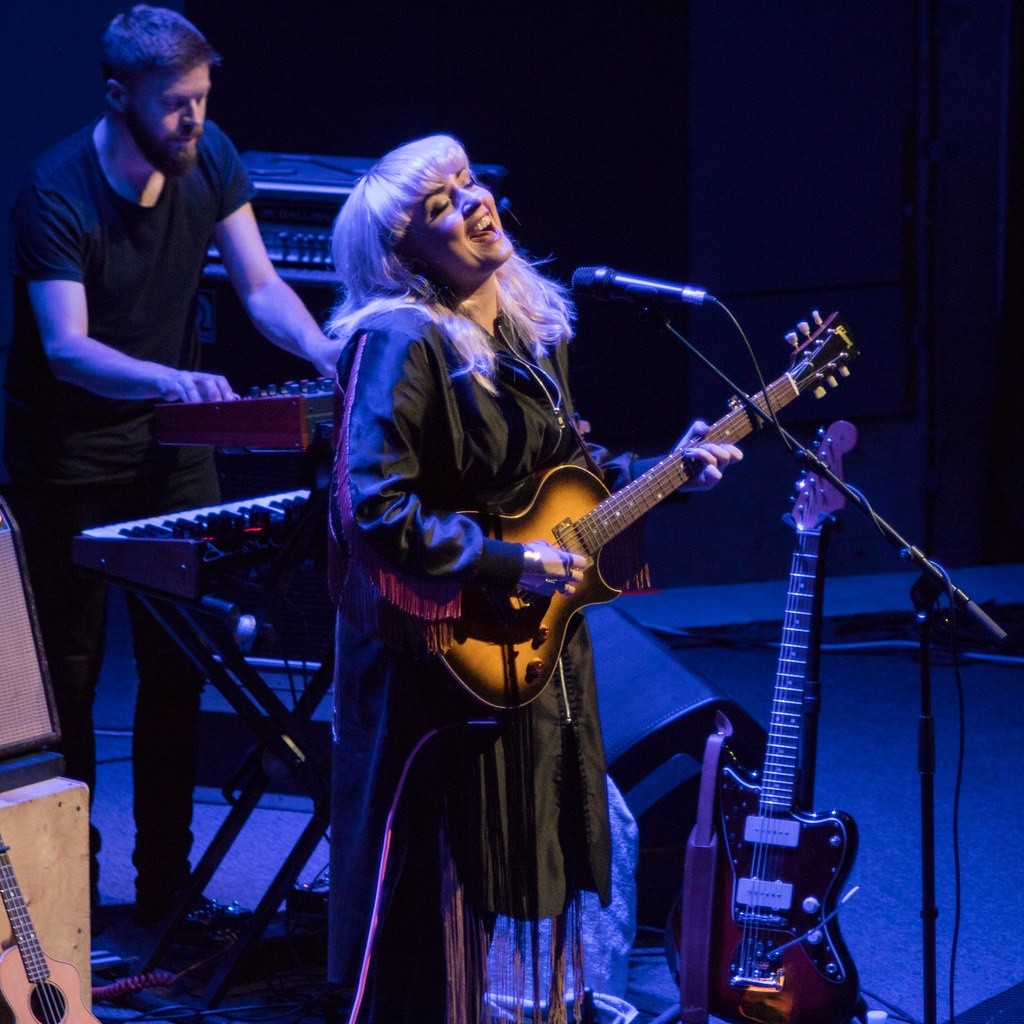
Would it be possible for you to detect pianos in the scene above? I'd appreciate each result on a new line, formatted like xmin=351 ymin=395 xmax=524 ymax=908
xmin=70 ymin=489 xmax=325 ymax=596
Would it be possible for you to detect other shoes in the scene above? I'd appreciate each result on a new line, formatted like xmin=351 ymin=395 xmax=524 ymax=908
xmin=138 ymin=884 xmax=251 ymax=942
xmin=90 ymin=934 xmax=140 ymax=969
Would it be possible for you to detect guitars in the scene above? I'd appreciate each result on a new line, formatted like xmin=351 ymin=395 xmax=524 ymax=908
xmin=0 ymin=842 xmax=101 ymax=1021
xmin=695 ymin=412 xmax=863 ymax=1024
xmin=405 ymin=309 xmax=865 ymax=713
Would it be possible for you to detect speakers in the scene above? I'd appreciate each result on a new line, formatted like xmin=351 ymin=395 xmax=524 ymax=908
xmin=584 ymin=605 xmax=768 ymax=950
xmin=0 ymin=498 xmax=61 ymax=760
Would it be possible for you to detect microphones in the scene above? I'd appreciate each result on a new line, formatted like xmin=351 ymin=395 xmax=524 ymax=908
xmin=570 ymin=266 xmax=718 ymax=306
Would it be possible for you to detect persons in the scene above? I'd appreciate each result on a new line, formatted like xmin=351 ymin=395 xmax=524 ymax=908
xmin=325 ymin=134 xmax=744 ymax=1024
xmin=1 ymin=4 xmax=350 ymax=951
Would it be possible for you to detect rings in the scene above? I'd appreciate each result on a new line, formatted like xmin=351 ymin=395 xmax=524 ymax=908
xmin=565 ymin=585 xmax=569 ymax=596
